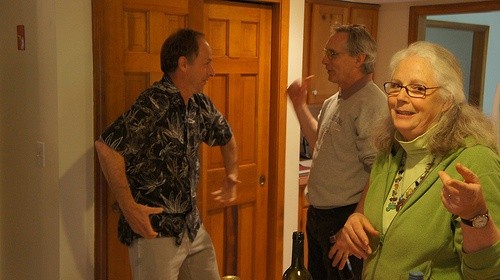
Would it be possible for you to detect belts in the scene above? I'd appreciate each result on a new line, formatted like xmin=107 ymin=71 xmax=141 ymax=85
xmin=310 ymin=205 xmax=357 ymax=216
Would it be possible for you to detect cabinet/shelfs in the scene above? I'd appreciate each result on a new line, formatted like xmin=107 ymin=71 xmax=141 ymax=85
xmin=301 ymin=0 xmax=381 ymax=107
xmin=298 ymin=174 xmax=309 ymax=270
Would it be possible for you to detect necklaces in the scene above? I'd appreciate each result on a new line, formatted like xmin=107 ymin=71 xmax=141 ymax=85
xmin=386 ymin=151 xmax=436 ymax=212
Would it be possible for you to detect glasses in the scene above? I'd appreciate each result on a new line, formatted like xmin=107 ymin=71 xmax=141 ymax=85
xmin=322 ymin=48 xmax=346 ymax=58
xmin=383 ymin=81 xmax=441 ymax=98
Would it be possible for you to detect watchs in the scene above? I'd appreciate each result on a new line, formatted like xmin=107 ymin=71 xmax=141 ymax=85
xmin=460 ymin=209 xmax=490 ymax=229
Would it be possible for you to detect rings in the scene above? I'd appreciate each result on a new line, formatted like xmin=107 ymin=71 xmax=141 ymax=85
xmin=447 ymin=194 xmax=450 ymax=200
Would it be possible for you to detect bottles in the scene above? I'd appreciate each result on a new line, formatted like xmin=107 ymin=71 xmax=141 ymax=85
xmin=282 ymin=231 xmax=312 ymax=280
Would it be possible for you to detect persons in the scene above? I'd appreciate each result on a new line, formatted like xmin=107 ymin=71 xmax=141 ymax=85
xmin=342 ymin=41 xmax=500 ymax=280
xmin=95 ymin=28 xmax=242 ymax=280
xmin=287 ymin=25 xmax=396 ymax=280
